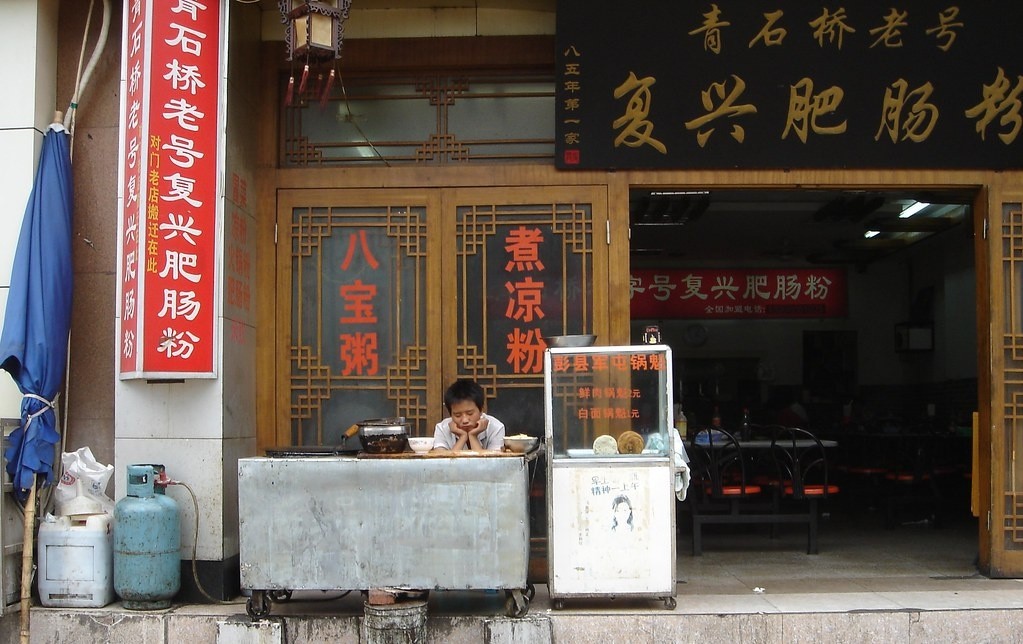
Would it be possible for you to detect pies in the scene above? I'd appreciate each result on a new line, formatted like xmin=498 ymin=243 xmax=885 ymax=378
xmin=592 ymin=435 xmax=617 ymax=456
xmin=617 ymin=431 xmax=645 ymax=454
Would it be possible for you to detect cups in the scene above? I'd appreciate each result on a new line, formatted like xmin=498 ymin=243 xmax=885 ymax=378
xmin=802 ymin=391 xmax=813 ymax=404
xmin=842 ymin=405 xmax=852 ymax=425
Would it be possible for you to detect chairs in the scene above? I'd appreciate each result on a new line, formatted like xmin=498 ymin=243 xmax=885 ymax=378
xmin=679 ymin=418 xmax=971 ymax=501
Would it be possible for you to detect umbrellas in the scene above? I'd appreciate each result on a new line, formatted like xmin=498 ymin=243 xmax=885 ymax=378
xmin=0 ymin=110 xmax=74 ymax=644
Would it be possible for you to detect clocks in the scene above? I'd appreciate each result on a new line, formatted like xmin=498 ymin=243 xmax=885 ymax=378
xmin=684 ymin=324 xmax=707 ymax=346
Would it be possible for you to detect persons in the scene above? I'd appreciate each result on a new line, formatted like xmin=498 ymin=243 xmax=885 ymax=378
xmin=433 ymin=378 xmax=505 ymax=451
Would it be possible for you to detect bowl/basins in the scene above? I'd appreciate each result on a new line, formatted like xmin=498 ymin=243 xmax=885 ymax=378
xmin=406 ymin=437 xmax=436 ymax=453
xmin=503 ymin=437 xmax=537 ymax=453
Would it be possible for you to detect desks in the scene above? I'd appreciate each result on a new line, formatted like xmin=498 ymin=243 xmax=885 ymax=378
xmin=683 ymin=439 xmax=838 ymax=555
xmin=830 ymin=429 xmax=972 ymax=530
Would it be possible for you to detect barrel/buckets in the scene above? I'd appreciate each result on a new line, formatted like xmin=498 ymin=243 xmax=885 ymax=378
xmin=36 ymin=510 xmax=113 ymax=607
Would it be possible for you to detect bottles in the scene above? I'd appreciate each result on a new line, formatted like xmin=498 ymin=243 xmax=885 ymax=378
xmin=926 ymin=398 xmax=937 ymax=418
xmin=957 ymin=399 xmax=976 ymax=425
xmin=740 ymin=407 xmax=753 ymax=442
xmin=675 ymin=411 xmax=687 ymax=440
xmin=687 ymin=412 xmax=698 ymax=438
xmin=711 ymin=406 xmax=721 ymax=429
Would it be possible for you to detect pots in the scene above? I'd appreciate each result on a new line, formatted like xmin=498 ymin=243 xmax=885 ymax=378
xmin=360 ymin=422 xmax=411 ymax=453
